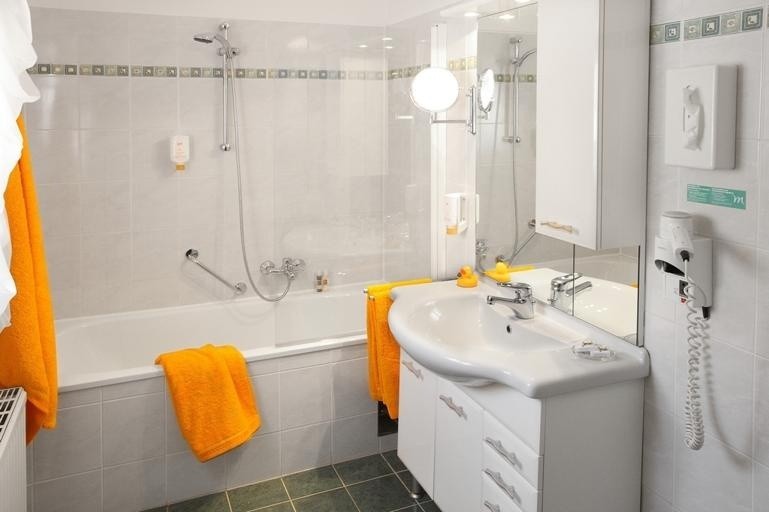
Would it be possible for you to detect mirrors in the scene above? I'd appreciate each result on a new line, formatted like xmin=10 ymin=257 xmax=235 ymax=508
xmin=409 ymin=66 xmax=469 ymax=125
xmin=475 ymin=1 xmax=645 ymax=346
xmin=477 ymin=69 xmax=494 ymax=121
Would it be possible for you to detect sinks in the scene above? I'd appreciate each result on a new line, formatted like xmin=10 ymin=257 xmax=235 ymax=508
xmin=388 ymin=275 xmax=650 ymax=399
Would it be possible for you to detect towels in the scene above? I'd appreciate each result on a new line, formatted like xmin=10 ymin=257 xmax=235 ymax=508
xmin=154 ymin=344 xmax=261 ymax=463
xmin=365 ymin=278 xmax=435 ymax=420
xmin=1 ymin=115 xmax=59 ymax=442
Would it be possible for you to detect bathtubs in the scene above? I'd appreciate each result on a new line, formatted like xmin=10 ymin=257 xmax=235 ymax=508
xmin=25 ymin=278 xmax=430 ymax=509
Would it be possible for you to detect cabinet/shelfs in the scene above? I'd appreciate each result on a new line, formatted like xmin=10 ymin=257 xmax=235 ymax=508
xmin=396 ymin=347 xmax=643 ymax=512
xmin=535 ymin=2 xmax=648 ymax=249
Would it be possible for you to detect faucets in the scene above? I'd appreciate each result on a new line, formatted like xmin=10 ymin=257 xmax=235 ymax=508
xmin=487 ymin=281 xmax=534 ymax=320
xmin=548 ymin=272 xmax=593 ymax=309
